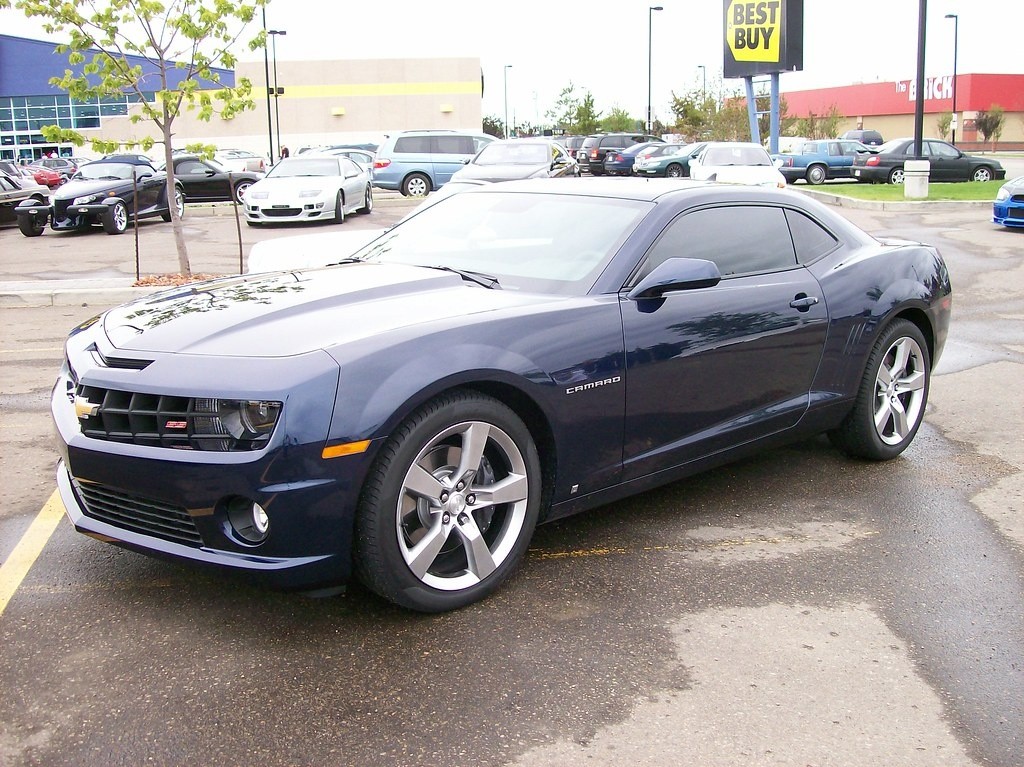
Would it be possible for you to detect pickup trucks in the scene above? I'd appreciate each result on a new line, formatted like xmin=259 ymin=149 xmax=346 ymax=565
xmin=770 ymin=139 xmax=871 ymax=185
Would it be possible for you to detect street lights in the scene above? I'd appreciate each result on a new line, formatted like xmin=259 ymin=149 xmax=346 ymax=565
xmin=504 ymin=64 xmax=513 ymax=138
xmin=945 ymin=12 xmax=959 ymax=146
xmin=648 ymin=7 xmax=664 ymax=135
xmin=697 ymin=65 xmax=706 ymax=104
xmin=267 ymin=27 xmax=288 ymax=160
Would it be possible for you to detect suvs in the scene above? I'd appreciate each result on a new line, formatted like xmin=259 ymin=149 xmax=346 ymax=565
xmin=372 ymin=128 xmax=502 ymax=199
xmin=838 ymin=130 xmax=884 ymax=145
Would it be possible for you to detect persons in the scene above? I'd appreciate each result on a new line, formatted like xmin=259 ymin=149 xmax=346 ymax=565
xmin=281 ymin=145 xmax=289 ymax=159
xmin=42 ymin=150 xmax=69 ymax=159
xmin=17 ymin=155 xmax=22 ymax=165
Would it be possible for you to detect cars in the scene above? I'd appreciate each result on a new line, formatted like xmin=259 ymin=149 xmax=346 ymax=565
xmin=49 ymin=172 xmax=954 ymax=615
xmin=243 ymin=155 xmax=373 ymax=225
xmin=991 ymin=172 xmax=1024 ymax=230
xmin=0 ymin=148 xmax=185 ymax=234
xmin=565 ymin=134 xmax=666 ymax=176
xmin=633 ymin=143 xmax=709 ymax=178
xmin=294 ymin=143 xmax=378 ymax=178
xmin=152 ymin=149 xmax=272 ymax=203
xmin=604 ymin=141 xmax=652 ymax=176
xmin=850 ymin=137 xmax=1005 ymax=185
xmin=688 ymin=140 xmax=785 ymax=188
